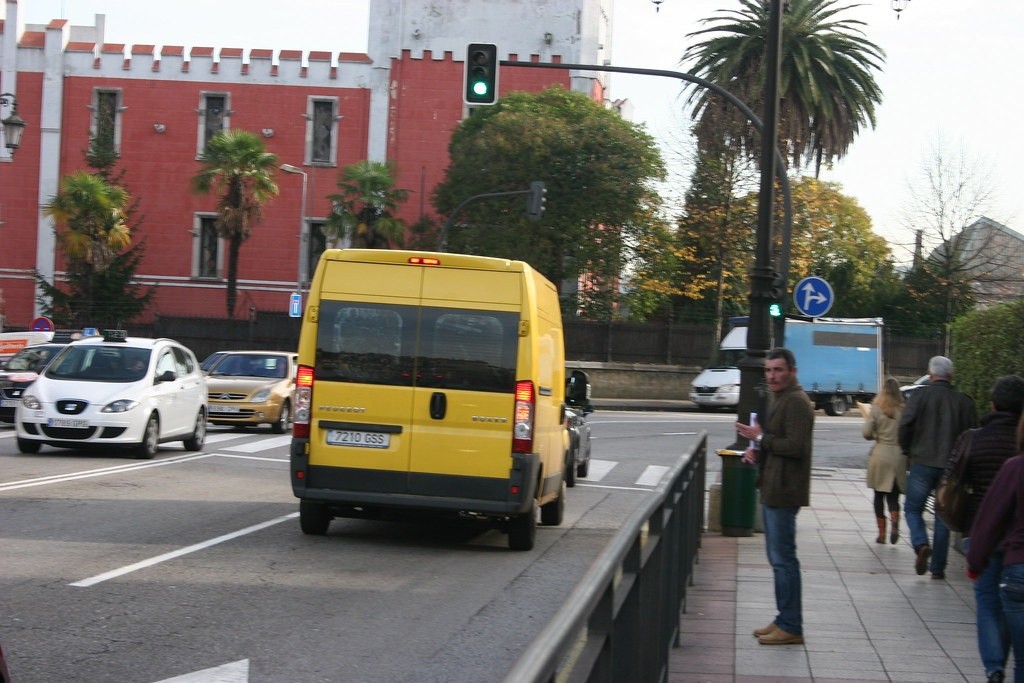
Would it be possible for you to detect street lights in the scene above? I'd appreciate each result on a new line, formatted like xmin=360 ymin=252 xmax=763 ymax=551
xmin=278 ymin=162 xmax=308 ymax=289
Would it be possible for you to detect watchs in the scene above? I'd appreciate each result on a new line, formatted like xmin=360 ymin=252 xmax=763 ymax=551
xmin=755 ymin=432 xmax=764 ymax=445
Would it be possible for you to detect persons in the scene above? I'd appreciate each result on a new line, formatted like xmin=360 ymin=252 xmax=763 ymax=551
xmin=937 ymin=375 xmax=1024 ymax=683
xmin=966 ymin=413 xmax=1024 ymax=683
xmin=863 ymin=378 xmax=909 ymax=544
xmin=735 ymin=348 xmax=813 ymax=645
xmin=899 ymin=356 xmax=976 ymax=580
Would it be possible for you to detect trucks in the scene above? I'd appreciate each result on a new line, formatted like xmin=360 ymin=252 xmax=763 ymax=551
xmin=689 ymin=315 xmax=883 ymax=416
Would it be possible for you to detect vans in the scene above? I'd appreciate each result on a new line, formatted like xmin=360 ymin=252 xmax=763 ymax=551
xmin=289 ymin=243 xmax=592 ymax=551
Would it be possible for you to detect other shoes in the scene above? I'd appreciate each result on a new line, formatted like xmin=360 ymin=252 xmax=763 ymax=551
xmin=988 ymin=672 xmax=1004 ymax=683
xmin=931 ymin=572 xmax=944 ymax=580
xmin=752 ymin=622 xmax=804 ymax=644
xmin=916 ymin=544 xmax=932 ymax=575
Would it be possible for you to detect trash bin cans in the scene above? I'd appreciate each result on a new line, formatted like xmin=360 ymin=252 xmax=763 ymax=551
xmin=715 ymin=450 xmax=756 ymax=537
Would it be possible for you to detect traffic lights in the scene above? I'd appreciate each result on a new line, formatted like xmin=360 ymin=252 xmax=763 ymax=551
xmin=463 ymin=41 xmax=499 ymax=105
xmin=528 ymin=181 xmax=547 ymax=221
xmin=770 ymin=296 xmax=784 ymax=319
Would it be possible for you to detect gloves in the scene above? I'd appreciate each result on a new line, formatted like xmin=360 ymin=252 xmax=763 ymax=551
xmin=966 ymin=564 xmax=982 ymax=578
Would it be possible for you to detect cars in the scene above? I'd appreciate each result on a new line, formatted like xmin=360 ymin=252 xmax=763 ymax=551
xmin=14 ymin=329 xmax=209 ymax=459
xmin=198 ymin=350 xmax=298 ymax=435
xmin=900 ymin=375 xmax=931 ymax=399
xmin=564 ymin=406 xmax=590 ymax=490
xmin=0 ymin=343 xmax=76 ymax=422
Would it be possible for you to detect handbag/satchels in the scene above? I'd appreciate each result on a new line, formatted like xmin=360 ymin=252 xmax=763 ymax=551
xmin=937 ymin=428 xmax=979 ymax=535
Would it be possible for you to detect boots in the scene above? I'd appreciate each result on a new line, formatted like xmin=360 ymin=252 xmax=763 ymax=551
xmin=890 ymin=510 xmax=900 ymax=544
xmin=876 ymin=516 xmax=887 ymax=544
